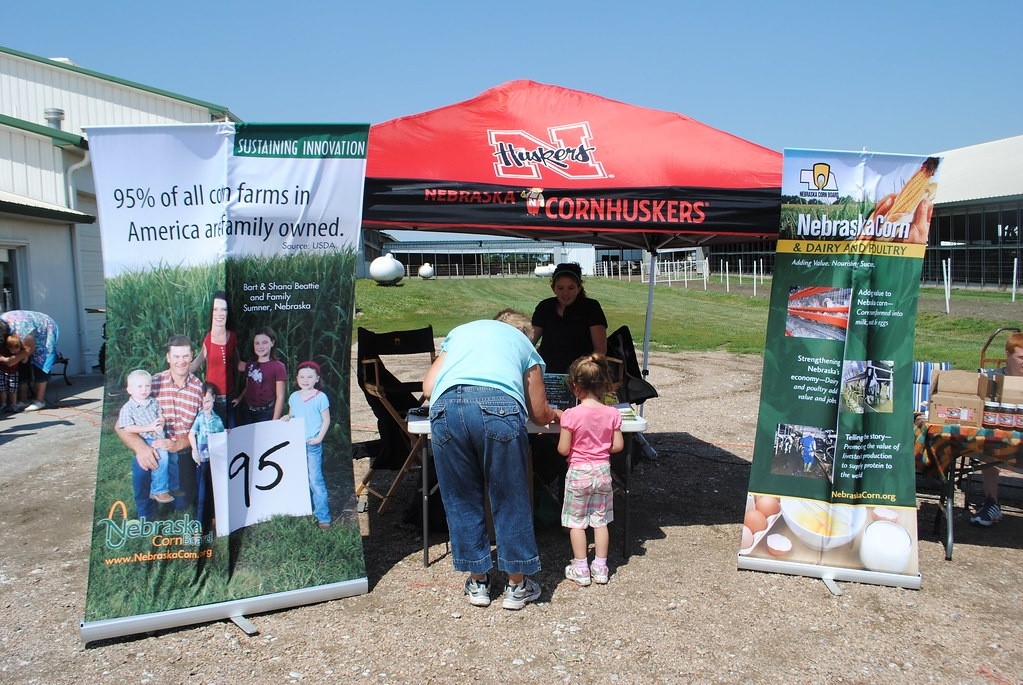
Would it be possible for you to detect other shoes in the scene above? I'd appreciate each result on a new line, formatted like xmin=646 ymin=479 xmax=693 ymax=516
xmin=170 ymin=488 xmax=186 ymax=497
xmin=149 ymin=493 xmax=175 ymax=503
xmin=564 ymin=560 xmax=591 ymax=586
xmin=319 ymin=522 xmax=333 ymax=531
xmin=589 ymin=560 xmax=609 ymax=584
xmin=24 ymin=400 xmax=46 ymax=411
xmin=17 ymin=401 xmax=33 ymax=410
xmin=0 ymin=404 xmax=9 ymax=410
xmin=804 ymin=470 xmax=811 ymax=473
xmin=11 ymin=403 xmax=19 ymax=411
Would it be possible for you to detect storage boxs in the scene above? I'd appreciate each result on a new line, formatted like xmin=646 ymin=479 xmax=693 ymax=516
xmin=928 ymin=368 xmax=1023 ymax=433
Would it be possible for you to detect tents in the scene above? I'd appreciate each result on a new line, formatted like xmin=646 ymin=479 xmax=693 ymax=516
xmin=360 ymin=77 xmax=782 ymax=433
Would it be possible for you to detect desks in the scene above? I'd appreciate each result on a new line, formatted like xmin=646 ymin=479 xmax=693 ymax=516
xmin=407 ymin=406 xmax=647 ymax=569
xmin=913 ymin=412 xmax=1023 ymax=563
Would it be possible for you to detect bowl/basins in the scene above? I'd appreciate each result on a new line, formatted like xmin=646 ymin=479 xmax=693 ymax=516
xmin=780 ymin=496 xmax=868 ymax=552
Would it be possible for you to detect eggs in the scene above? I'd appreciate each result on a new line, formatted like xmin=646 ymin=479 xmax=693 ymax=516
xmin=741 ymin=494 xmax=781 ymax=551
xmin=792 ymin=509 xmax=842 ymax=536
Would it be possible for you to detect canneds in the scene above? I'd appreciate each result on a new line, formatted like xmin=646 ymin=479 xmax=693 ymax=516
xmin=982 ymin=401 xmax=1023 ymax=432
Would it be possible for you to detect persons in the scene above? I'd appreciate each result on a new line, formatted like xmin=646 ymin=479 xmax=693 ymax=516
xmin=528 ymin=263 xmax=607 ymax=373
xmin=113 ymin=293 xmax=330 ymax=548
xmin=0 ymin=310 xmax=58 ymax=410
xmin=556 ymin=353 xmax=624 ymax=586
xmin=0 ymin=334 xmax=30 ymax=413
xmin=970 ymin=332 xmax=1023 ymax=526
xmin=420 ymin=308 xmax=564 ymax=613
xmin=800 ymin=432 xmax=816 ymax=473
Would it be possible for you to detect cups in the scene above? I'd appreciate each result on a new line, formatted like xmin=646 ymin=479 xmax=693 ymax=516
xmin=859 ymin=520 xmax=912 ymax=574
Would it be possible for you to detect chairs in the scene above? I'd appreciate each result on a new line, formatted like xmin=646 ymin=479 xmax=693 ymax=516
xmin=32 ymin=352 xmax=72 ymax=396
xmin=355 ymin=325 xmax=658 ymax=516
xmin=913 ymin=361 xmax=955 ymax=505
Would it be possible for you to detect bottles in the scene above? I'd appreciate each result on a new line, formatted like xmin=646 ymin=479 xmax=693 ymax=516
xmin=982 ymin=402 xmax=1023 ymax=433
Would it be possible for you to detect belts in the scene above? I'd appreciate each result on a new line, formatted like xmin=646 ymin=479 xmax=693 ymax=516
xmin=247 ymin=401 xmax=274 ymax=411
xmin=214 ymin=394 xmax=236 ymax=399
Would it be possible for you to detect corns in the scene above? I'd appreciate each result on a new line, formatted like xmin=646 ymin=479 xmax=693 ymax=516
xmin=880 ymin=156 xmax=940 ymax=242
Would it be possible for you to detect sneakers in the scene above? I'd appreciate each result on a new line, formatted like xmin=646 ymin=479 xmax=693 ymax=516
xmin=503 ymin=576 xmax=541 ymax=610
xmin=464 ymin=572 xmax=492 ymax=606
xmin=970 ymin=497 xmax=1002 ymax=525
